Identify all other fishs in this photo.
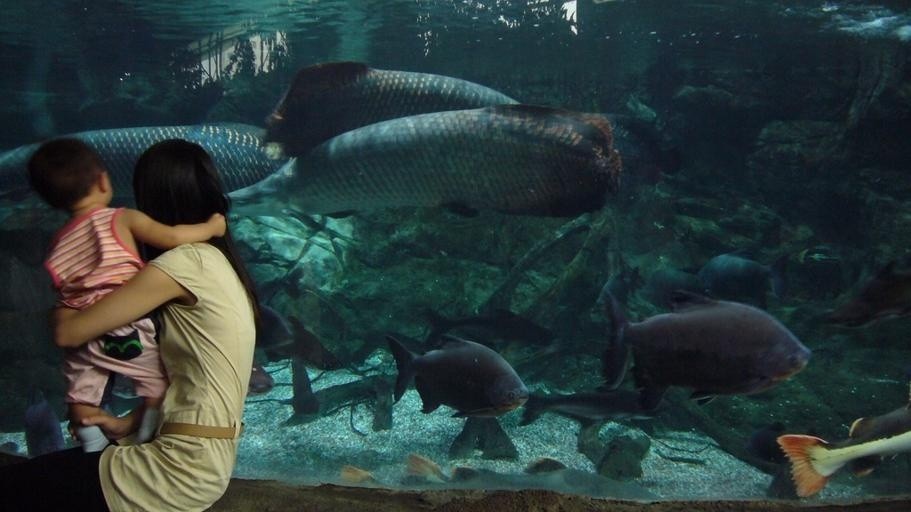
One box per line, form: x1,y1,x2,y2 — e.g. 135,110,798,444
0,60,910,501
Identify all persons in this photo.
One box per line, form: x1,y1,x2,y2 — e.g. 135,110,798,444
27,135,226,453
0,135,260,511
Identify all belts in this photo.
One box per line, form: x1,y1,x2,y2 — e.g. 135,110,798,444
161,422,244,439
